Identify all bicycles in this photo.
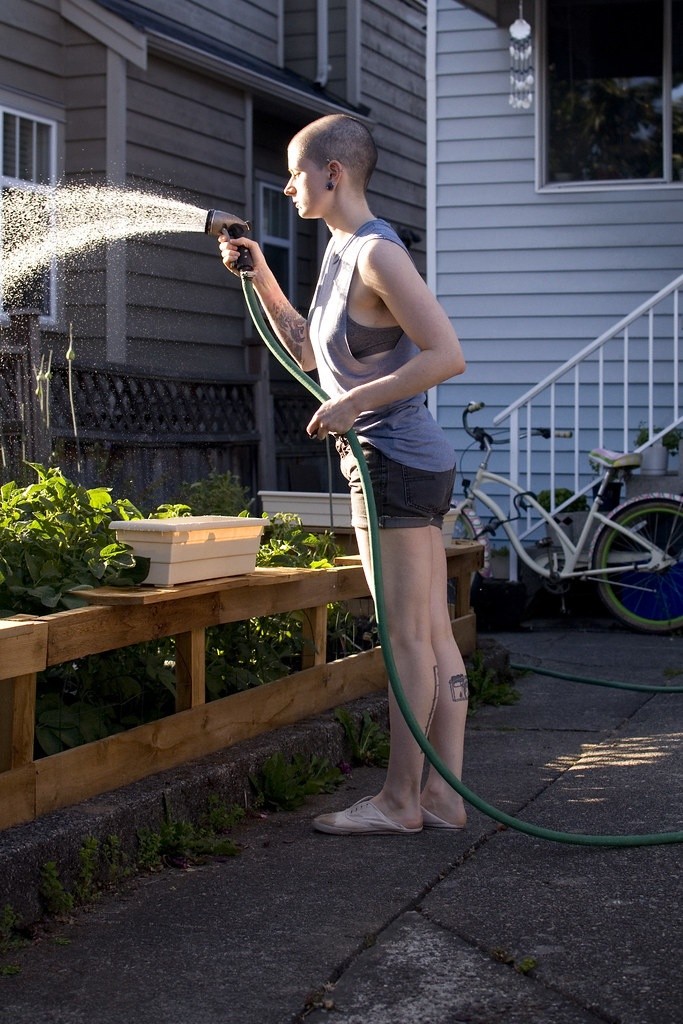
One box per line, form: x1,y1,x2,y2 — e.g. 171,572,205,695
440,402,683,635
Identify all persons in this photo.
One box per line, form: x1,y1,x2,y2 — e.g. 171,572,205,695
218,114,468,835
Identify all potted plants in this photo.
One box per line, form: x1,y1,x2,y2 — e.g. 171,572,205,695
538,488,606,546
634,421,683,476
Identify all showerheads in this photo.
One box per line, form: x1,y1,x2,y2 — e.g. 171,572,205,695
204,208,255,272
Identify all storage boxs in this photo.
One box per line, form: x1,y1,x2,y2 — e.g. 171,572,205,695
257,490,353,527
106,511,271,589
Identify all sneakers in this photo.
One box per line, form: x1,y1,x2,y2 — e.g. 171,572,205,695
312,796,424,834
420,804,467,831
